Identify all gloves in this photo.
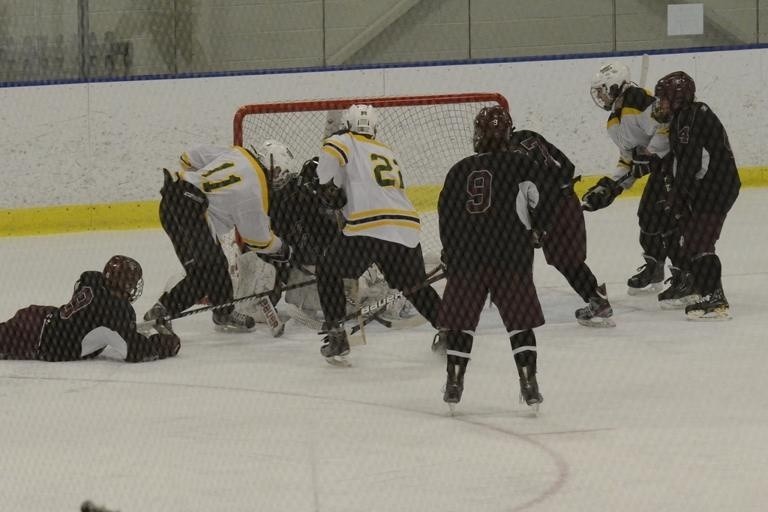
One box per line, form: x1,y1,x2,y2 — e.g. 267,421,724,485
583,176,623,211
630,144,662,178
269,244,294,263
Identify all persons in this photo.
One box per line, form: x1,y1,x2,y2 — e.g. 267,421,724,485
581,59,700,300
510,131,613,321
142,137,295,327
223,157,349,323
1,255,181,363
651,70,741,311
303,103,443,358
438,105,545,405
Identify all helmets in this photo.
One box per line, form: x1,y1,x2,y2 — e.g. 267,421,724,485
105,255,144,304
472,106,513,151
339,103,374,136
651,71,695,123
590,60,630,113
255,141,301,191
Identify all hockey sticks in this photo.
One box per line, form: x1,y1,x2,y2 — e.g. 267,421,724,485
293,273,445,330
293,256,428,329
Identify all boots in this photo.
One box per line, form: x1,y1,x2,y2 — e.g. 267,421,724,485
443,362,465,402
213,307,255,328
518,365,543,405
141,292,182,336
321,320,350,357
576,283,613,319
628,254,729,318
